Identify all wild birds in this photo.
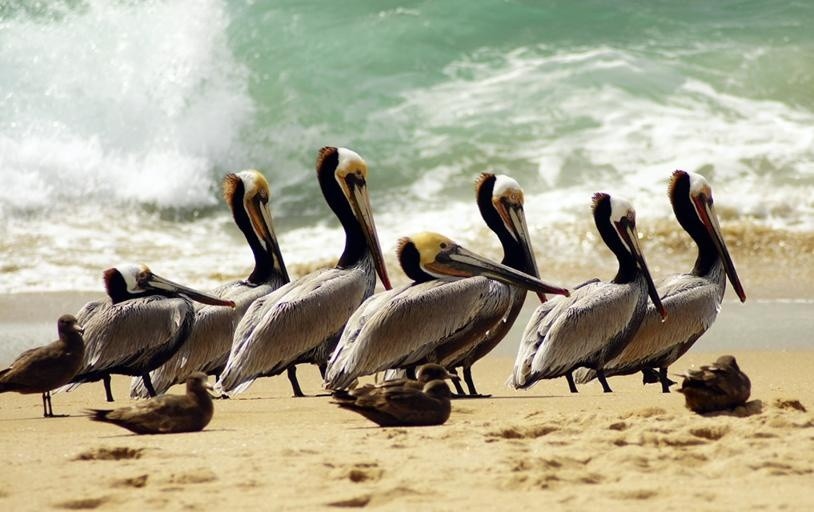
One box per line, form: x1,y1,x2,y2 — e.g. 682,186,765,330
674,351,753,417
323,229,570,392
325,361,462,427
502,190,669,394
0,311,87,421
79,370,215,433
49,260,235,403
571,167,750,392
214,143,395,401
128,167,293,401
417,169,549,401
